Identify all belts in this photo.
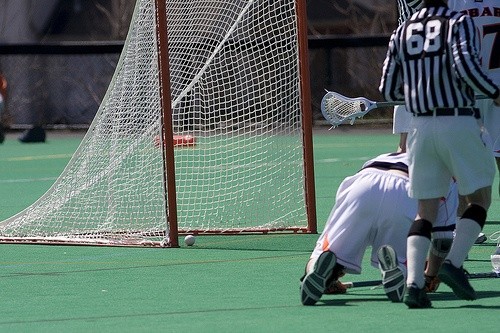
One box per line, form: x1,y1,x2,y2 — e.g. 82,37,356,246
413,107,481,118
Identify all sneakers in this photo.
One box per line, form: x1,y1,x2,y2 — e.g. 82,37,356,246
403,283,433,308
438,259,477,300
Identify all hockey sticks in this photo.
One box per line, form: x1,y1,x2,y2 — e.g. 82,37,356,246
321,88,500,128
338,271,500,289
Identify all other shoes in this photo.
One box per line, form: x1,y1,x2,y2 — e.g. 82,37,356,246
474,233,487,244
376,245,406,304
301,251,337,306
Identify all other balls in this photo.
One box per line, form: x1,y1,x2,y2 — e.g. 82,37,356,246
184,236,195,246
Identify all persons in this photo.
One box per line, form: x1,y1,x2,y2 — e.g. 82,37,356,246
298,0,500,307
